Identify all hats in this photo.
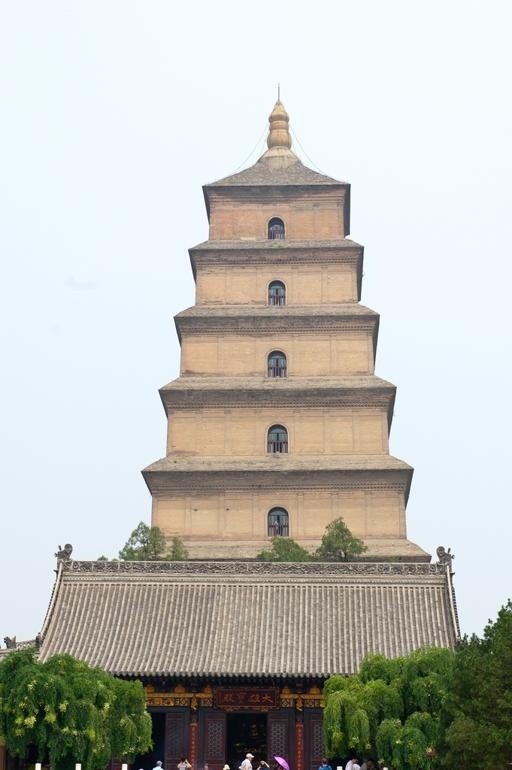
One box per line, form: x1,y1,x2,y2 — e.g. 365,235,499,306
246,753,255,758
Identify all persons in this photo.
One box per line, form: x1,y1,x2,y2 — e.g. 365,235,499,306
152,752,388,770
